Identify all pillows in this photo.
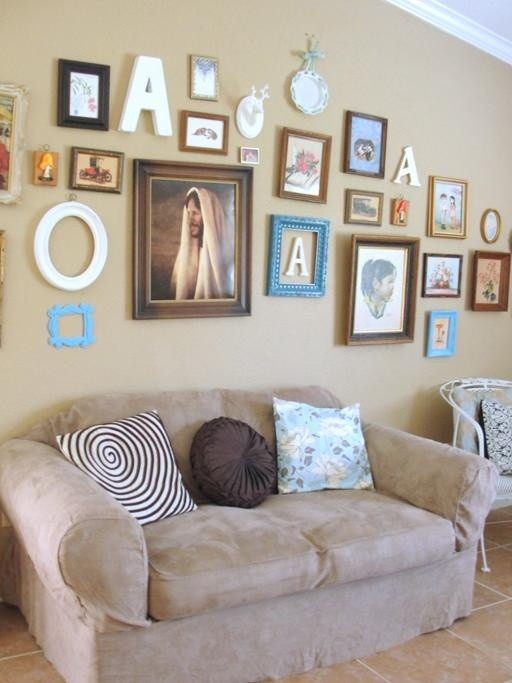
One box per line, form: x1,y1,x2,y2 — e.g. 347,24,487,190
480,399,512,475
189,416,276,508
270,397,375,495
55,409,198,526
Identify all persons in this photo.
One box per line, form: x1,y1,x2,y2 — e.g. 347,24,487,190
361,259,397,320
439,194,471,229
166,185,235,299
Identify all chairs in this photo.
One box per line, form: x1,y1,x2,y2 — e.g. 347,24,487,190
440,378,512,572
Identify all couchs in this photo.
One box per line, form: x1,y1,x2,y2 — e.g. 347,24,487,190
0,384,498,683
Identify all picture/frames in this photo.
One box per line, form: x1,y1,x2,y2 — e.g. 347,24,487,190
187,52,222,104
55,56,112,135
128,156,256,321
425,173,470,242
342,187,385,228
470,248,510,313
237,145,262,165
68,145,126,194
420,251,464,299
423,309,459,357
177,108,232,156
341,108,390,180
343,232,422,348
276,124,334,206
264,212,334,299
0,79,34,204
